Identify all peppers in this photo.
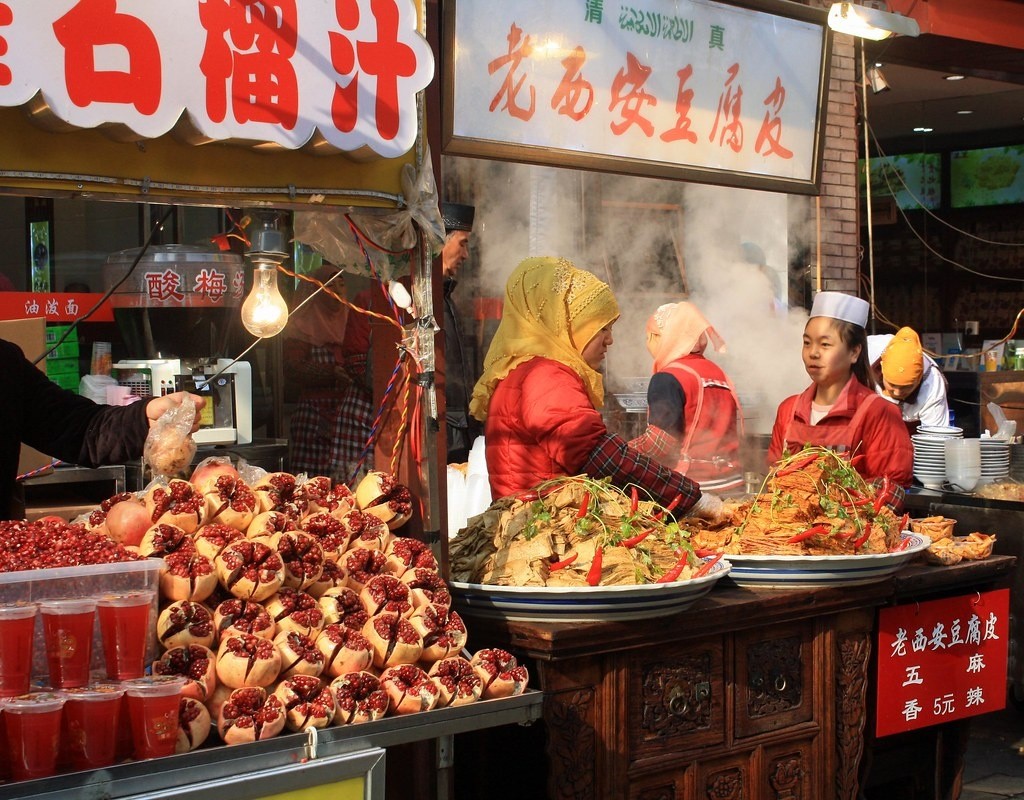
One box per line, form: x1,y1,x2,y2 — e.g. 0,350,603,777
775,452,911,553
513,480,724,582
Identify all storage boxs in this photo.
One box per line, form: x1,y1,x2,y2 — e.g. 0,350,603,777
0,548,165,679
45,326,79,395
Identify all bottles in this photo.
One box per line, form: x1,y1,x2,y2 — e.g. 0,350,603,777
1013,347,1024,372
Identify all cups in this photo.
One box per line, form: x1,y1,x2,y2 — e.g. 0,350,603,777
89,341,113,376
0,590,189,782
106,385,132,406
983,350,997,373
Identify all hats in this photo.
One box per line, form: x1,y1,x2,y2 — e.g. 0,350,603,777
878,326,925,385
807,290,871,330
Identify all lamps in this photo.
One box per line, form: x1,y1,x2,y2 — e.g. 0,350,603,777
827,0,921,42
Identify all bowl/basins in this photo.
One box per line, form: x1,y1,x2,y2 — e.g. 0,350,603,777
910,425,1011,494
907,516,997,567
612,375,651,414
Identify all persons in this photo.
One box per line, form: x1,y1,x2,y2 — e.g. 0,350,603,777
865,326,949,436
765,291,914,516
626,300,746,501
282,263,374,492
440,202,484,465
469,256,740,525
0,337,207,521
0,273,129,377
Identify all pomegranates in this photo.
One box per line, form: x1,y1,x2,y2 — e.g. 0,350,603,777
0,427,528,754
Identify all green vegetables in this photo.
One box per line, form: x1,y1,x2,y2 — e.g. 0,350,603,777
522,473,695,583
734,440,891,550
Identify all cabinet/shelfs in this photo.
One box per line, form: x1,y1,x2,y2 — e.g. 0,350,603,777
940,368,1024,437
460,556,1018,800
855,132,1024,336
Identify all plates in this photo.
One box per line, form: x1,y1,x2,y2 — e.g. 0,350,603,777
449,555,733,623
714,529,933,590
